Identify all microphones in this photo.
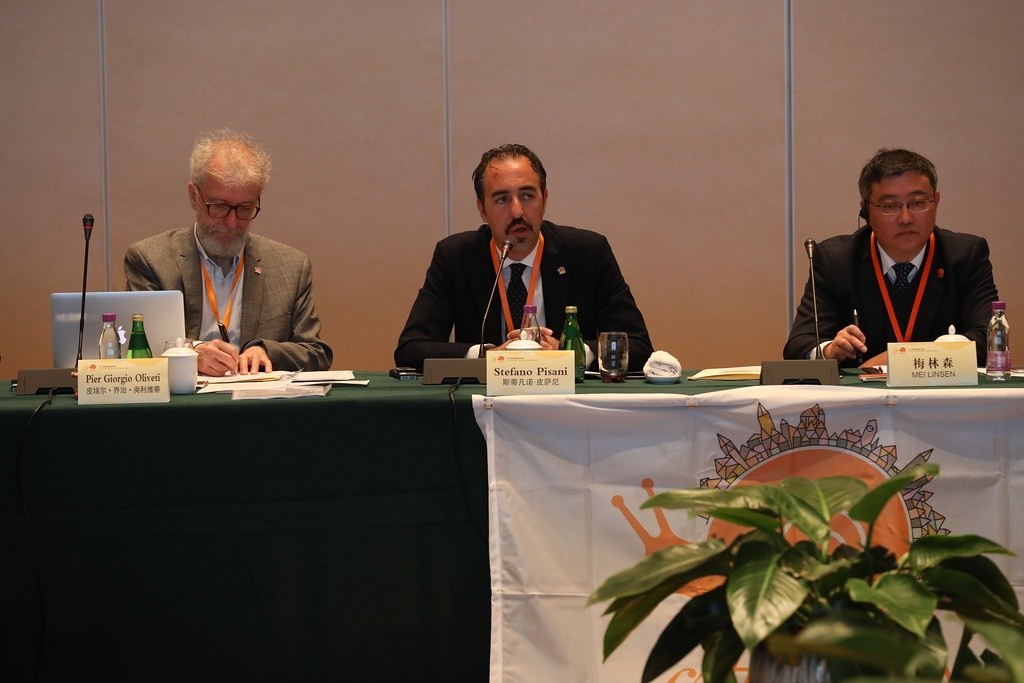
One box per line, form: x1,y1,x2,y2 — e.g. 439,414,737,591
478,239,513,358
805,238,824,360
76,213,94,369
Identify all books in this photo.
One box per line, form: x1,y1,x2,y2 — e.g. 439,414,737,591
859,365,887,382
196,370,371,400
687,366,761,380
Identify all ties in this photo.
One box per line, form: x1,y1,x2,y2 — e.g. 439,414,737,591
505,261,528,341
891,261,915,295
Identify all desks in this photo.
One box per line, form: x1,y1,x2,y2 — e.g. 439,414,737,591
1,370,1024,683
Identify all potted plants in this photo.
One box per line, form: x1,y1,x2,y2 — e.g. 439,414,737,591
580,463,1024,683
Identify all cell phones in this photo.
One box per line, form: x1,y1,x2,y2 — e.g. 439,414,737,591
390,369,419,380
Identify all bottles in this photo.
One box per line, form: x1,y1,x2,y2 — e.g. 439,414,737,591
518,305,541,345
985,301,1012,380
557,305,586,384
125,313,152,358
98,313,121,359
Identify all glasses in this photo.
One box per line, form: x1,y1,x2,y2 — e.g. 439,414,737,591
867,195,935,215
194,183,261,220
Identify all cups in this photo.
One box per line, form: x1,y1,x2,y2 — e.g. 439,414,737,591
933,325,971,343
505,330,542,351
161,338,199,394
597,331,629,383
164,337,193,351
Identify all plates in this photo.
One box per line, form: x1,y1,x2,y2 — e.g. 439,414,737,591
646,375,681,384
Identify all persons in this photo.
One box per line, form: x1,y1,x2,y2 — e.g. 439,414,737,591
393,145,654,376
782,149,999,368
123,127,333,377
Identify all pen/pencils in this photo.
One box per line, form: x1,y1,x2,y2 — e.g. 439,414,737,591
218,323,237,374
853,309,863,363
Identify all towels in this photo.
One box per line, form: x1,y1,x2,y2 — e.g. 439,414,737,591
642,349,682,377
